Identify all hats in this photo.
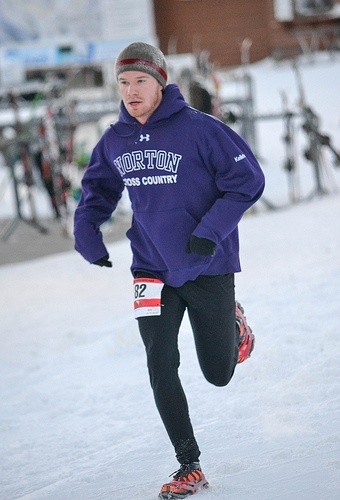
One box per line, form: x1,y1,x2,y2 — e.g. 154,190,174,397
115,42,168,89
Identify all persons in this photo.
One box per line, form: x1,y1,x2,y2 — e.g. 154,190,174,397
72,43,265,500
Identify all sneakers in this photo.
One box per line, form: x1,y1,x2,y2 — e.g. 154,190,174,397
235,300,254,363
159,462,208,499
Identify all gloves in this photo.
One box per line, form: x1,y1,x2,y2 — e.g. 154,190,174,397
94,255,112,268
185,236,216,256
